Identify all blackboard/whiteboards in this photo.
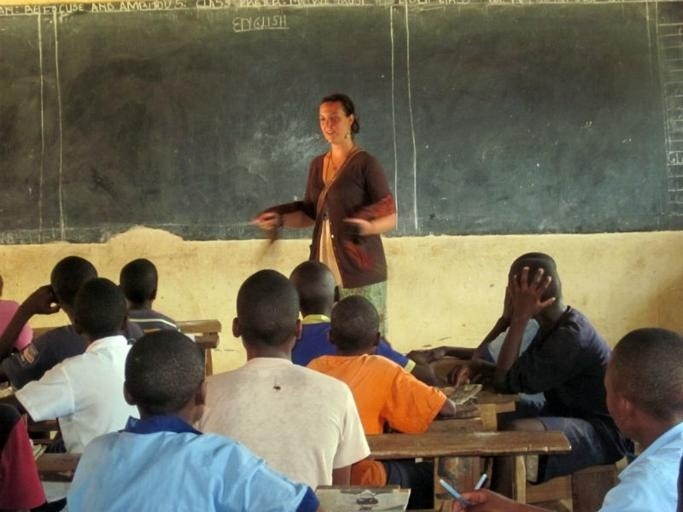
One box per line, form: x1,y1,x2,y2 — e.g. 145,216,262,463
0,0,681,245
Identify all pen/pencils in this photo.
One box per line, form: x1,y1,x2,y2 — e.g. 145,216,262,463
434,476,461,498
473,471,491,491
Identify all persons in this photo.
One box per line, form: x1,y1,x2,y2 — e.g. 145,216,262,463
60,328,325,512
189,268,372,490
1,255,147,391
11,277,141,454
438,327,683,512
449,252,632,485
426,250,559,367
252,93,398,339
303,295,457,509
120,257,183,344
1,276,34,351
0,402,52,511
289,259,440,387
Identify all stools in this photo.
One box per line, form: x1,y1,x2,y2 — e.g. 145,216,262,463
574,455,628,497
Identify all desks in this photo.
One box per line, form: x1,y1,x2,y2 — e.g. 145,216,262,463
30,327,59,350
364,433,572,504
0,382,63,446
436,385,513,433
35,453,82,482
310,485,412,512
175,320,222,380
